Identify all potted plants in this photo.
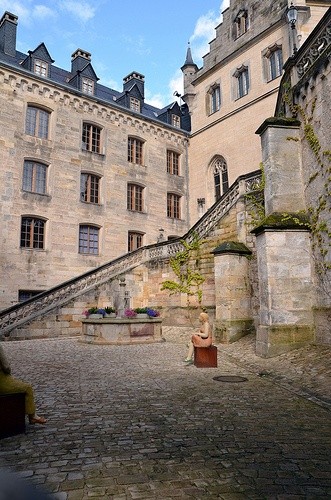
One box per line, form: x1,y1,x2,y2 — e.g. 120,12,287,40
104,306,116,319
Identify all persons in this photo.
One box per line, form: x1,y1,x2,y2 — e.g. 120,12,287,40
0,342,47,425
183,313,212,362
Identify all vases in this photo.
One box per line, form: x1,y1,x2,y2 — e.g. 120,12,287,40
136,313,149,319
89,314,103,319
150,315,159,319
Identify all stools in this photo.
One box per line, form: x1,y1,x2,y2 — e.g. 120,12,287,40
0,390,28,437
194,344,217,368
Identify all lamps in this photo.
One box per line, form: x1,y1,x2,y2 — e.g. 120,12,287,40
286,1,298,30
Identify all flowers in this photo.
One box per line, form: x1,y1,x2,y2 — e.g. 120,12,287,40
83,307,105,316
117,307,160,317
